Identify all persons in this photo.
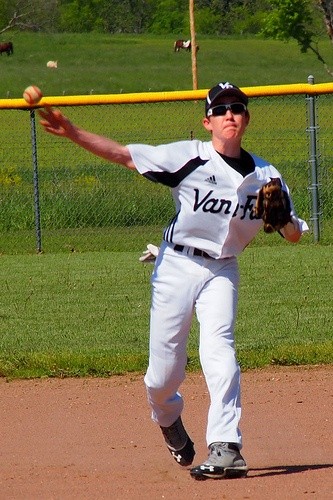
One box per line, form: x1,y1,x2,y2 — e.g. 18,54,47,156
38,81,310,482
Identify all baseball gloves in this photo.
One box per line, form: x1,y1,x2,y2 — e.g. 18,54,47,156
253,178,291,233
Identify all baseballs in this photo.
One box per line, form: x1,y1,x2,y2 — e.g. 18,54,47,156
23,86,42,104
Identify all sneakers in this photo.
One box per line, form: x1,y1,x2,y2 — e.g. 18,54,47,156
190,442,248,478
158,416,194,467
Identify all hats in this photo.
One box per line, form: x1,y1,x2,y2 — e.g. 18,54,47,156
205,81,248,115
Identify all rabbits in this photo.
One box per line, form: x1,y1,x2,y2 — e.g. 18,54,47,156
46,55,59,69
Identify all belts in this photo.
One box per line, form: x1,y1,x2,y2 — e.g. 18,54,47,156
174,244,209,258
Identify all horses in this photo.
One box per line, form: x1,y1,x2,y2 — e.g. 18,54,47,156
0,41,14,56
174,39,191,53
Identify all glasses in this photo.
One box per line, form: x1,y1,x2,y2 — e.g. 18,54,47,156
207,103,247,117
